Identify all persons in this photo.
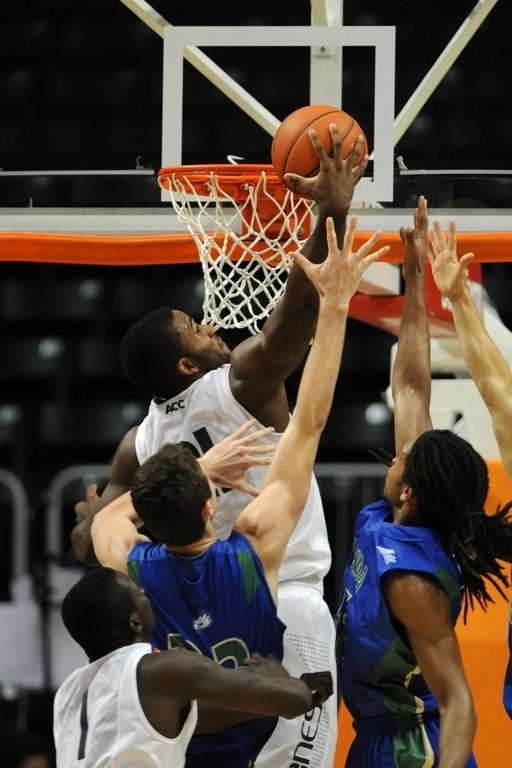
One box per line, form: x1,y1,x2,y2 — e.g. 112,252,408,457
423,214,511,717
70,123,371,767
90,214,390,768
52,566,313,768
334,192,511,768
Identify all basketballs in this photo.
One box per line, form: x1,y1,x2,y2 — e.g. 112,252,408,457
271,105,368,199
